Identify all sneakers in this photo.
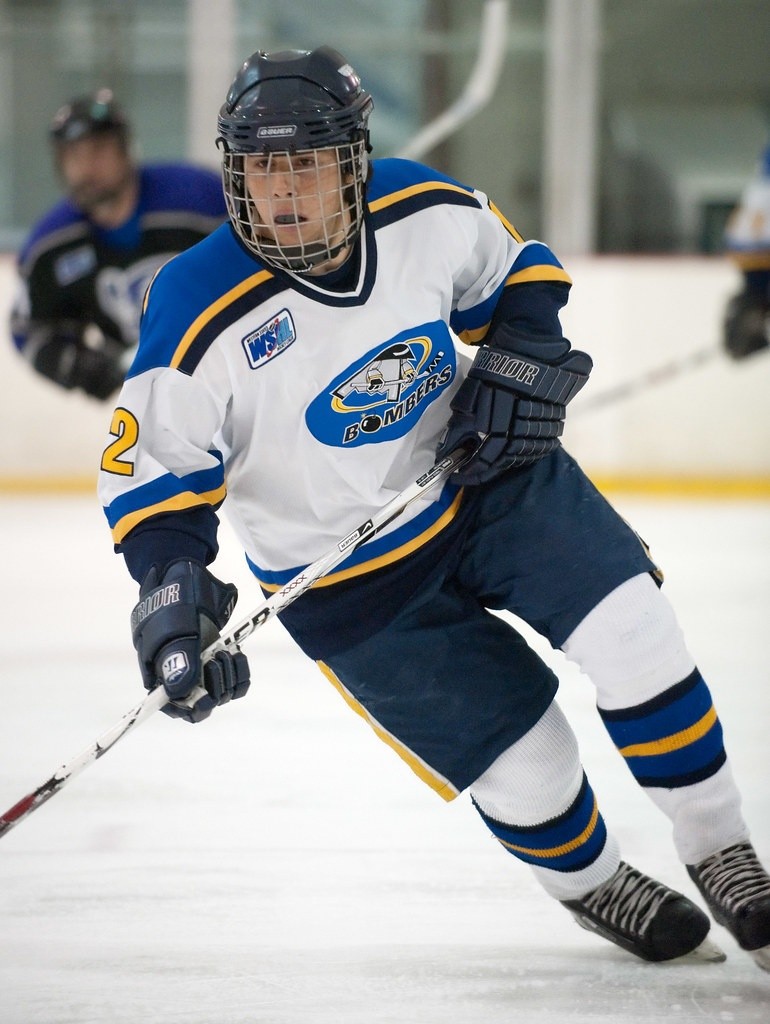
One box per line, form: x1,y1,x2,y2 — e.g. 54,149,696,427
558,860,729,964
685,839,769,974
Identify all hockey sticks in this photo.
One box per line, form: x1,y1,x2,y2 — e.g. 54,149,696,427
567,337,727,421
0,431,488,839
118,0,507,371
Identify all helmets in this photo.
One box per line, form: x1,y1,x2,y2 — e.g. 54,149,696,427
214,43,375,275
47,89,135,161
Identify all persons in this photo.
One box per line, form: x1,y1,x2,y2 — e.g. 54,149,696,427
10,93,230,401
98,45,770,969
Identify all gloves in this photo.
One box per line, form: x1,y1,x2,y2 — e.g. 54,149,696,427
431,327,593,487
130,559,252,725
